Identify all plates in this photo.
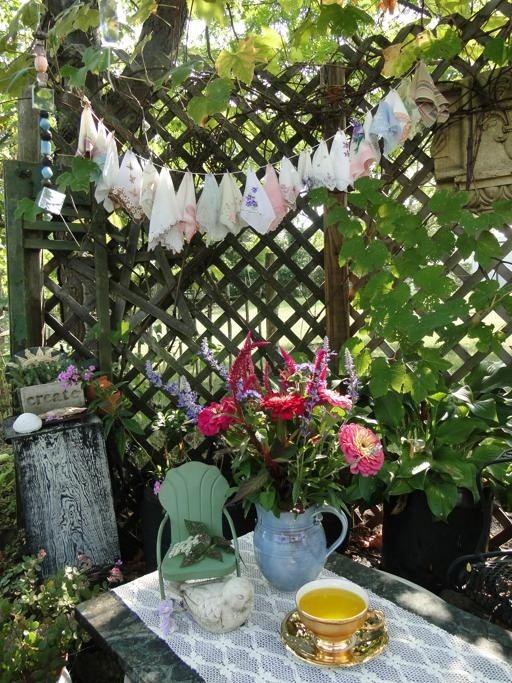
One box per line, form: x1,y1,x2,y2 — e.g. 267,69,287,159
281,608,389,668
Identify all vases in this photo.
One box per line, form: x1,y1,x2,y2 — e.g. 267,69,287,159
13,379,84,415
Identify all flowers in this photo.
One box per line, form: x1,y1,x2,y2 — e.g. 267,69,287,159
139,336,386,507
6,346,67,388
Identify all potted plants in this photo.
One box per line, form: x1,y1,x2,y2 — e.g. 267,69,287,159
303,168,512,591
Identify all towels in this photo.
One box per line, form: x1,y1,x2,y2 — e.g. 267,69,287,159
73,60,457,252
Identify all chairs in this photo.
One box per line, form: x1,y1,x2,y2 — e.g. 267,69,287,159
443,549,511,632
148,459,247,604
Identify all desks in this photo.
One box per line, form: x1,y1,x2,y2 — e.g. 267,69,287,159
71,528,512,683
2,410,123,583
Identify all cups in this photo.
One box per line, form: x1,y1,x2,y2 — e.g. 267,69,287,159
295,577,386,655
